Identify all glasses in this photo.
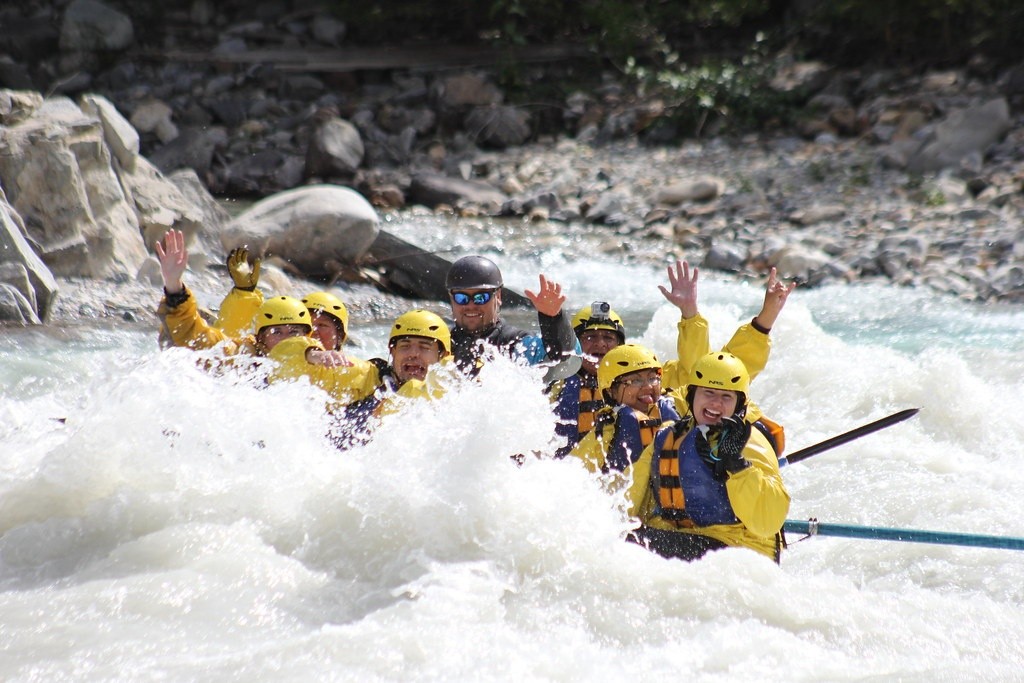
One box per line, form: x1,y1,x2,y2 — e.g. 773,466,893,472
450,289,498,305
614,376,662,387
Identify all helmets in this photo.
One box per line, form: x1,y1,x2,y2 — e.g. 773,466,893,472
685,352,749,416
388,311,452,368
597,345,663,405
446,256,503,289
255,296,313,337
301,292,347,346
571,306,625,345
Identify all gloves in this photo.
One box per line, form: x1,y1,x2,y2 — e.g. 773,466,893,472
716,413,752,474
227,245,260,291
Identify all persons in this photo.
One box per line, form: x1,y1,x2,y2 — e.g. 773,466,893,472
211,245,349,351
609,350,791,564
155,228,313,384
445,255,584,383
545,260,710,475
264,308,470,452
562,266,796,527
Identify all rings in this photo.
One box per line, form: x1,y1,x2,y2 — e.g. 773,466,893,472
553,290,561,296
